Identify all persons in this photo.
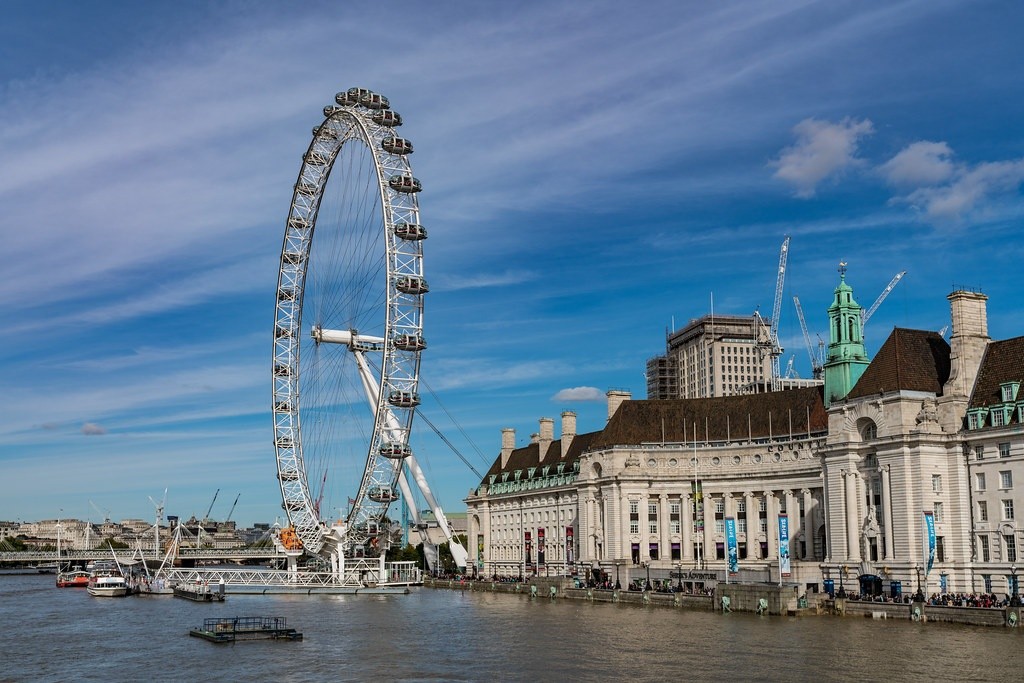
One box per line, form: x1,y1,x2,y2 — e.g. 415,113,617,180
825,591,1024,611
205,579,209,593
436,573,529,582
574,580,715,595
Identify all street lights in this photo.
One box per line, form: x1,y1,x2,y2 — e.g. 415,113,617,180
836,562,847,599
1010,563,1022,607
674,563,683,592
913,563,924,602
645,563,652,591
615,561,622,589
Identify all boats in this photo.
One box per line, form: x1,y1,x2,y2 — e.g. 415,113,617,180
56,565,90,589
88,561,127,598
